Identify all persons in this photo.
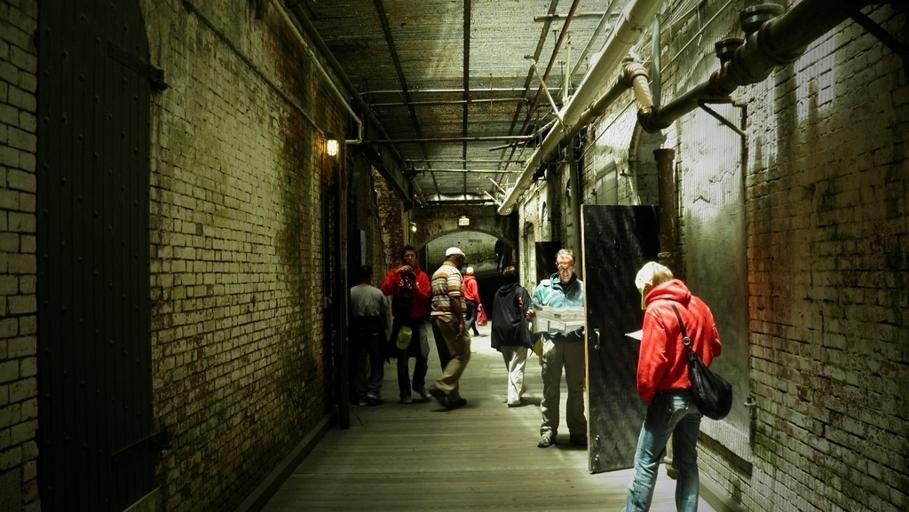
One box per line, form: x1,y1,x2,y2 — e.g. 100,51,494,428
381,246,432,404
527,247,587,447
463,267,481,337
623,261,723,511
350,263,390,406
492,263,542,408
431,247,474,408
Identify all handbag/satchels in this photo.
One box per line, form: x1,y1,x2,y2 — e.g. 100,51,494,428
477,306,487,325
684,335,732,420
515,319,534,347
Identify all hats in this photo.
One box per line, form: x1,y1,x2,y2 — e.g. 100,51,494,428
635,261,662,310
445,247,465,256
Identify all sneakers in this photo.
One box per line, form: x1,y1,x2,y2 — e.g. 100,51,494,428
402,396,412,403
537,430,556,447
359,389,377,405
430,387,451,407
412,385,432,399
450,397,467,409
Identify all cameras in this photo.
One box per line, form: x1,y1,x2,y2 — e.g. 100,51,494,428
401,270,416,286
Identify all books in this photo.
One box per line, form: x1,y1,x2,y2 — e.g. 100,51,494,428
623,329,643,342
